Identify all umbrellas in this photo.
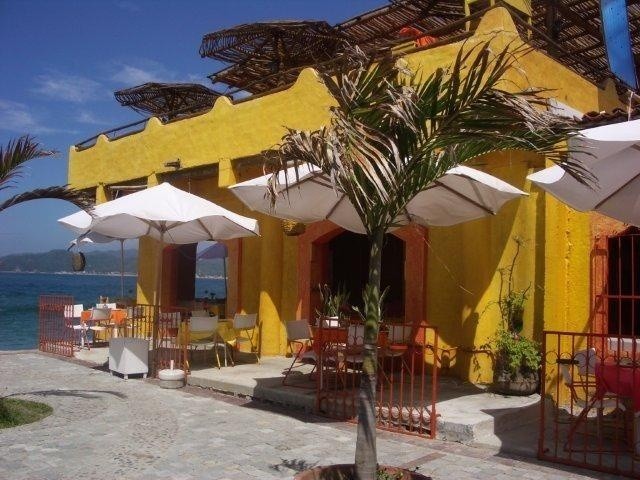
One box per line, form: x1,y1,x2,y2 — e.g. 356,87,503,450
65,230,129,304
57,182,260,378
196,241,229,297
525,118,640,228
227,150,529,235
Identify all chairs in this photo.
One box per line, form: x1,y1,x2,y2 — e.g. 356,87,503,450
156,310,260,370
63,298,144,351
557,346,627,453
281,314,416,394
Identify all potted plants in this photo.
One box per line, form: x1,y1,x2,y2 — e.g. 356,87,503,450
467,232,544,396
